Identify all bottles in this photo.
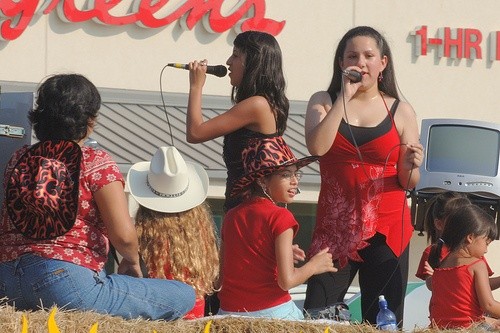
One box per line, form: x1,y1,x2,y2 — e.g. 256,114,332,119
376,296,396,333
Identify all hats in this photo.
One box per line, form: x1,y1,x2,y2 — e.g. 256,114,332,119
7,140,82,239
127,146,209,213
227,136,322,197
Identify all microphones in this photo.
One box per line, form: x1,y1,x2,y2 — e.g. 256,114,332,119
168,63,227,77
342,69,361,82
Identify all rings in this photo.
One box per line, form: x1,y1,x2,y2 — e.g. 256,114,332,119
199,62,208,66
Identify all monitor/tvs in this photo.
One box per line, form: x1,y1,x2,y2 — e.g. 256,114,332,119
415,118,500,200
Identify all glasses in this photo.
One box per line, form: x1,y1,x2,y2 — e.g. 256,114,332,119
270,169,303,179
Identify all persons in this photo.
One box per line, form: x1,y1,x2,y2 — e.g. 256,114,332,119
0,73,196,321
186,31,289,214
117,144,224,320
428,203,500,331
303,25,424,333
216,135,338,321
415,190,500,292
